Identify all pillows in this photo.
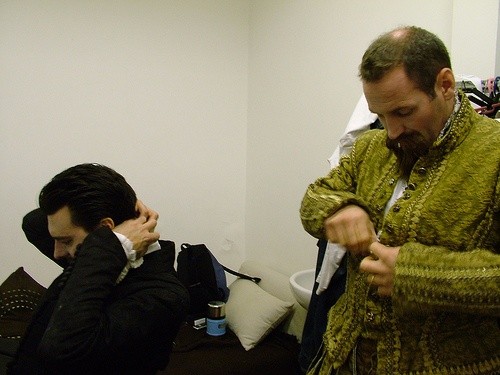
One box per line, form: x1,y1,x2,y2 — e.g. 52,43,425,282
222,270,295,351
0,267,48,358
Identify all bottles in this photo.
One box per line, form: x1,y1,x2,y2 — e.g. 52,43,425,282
206,301,227,336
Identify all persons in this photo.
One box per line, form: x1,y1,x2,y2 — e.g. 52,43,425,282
298,26,500,375
3,162,188,375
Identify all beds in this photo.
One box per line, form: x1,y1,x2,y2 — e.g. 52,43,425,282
0,307,302,375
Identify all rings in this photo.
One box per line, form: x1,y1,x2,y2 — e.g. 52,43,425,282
371,273,375,284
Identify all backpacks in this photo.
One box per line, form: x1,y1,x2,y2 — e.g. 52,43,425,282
177,243,262,314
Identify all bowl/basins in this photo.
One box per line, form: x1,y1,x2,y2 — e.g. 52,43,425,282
290,270,315,308
